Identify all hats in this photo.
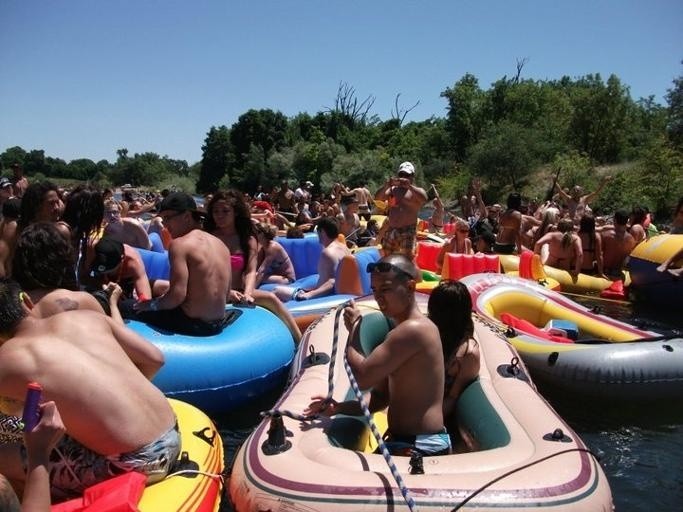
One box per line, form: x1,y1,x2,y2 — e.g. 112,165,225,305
90,239,125,272
159,192,208,218
121,184,132,188
397,161,415,177
0,177,13,189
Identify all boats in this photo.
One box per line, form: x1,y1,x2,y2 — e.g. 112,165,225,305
459,271,683,403
231,290,614,511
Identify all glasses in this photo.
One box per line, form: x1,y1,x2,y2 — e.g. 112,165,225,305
367,262,414,279
399,172,411,177
107,210,120,215
161,210,185,225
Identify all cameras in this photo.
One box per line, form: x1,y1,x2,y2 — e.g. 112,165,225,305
391,177,400,186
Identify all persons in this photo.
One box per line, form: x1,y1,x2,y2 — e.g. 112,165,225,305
1,160,682,512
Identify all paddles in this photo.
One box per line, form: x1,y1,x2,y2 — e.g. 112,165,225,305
373,200,387,210
545,166,561,202
